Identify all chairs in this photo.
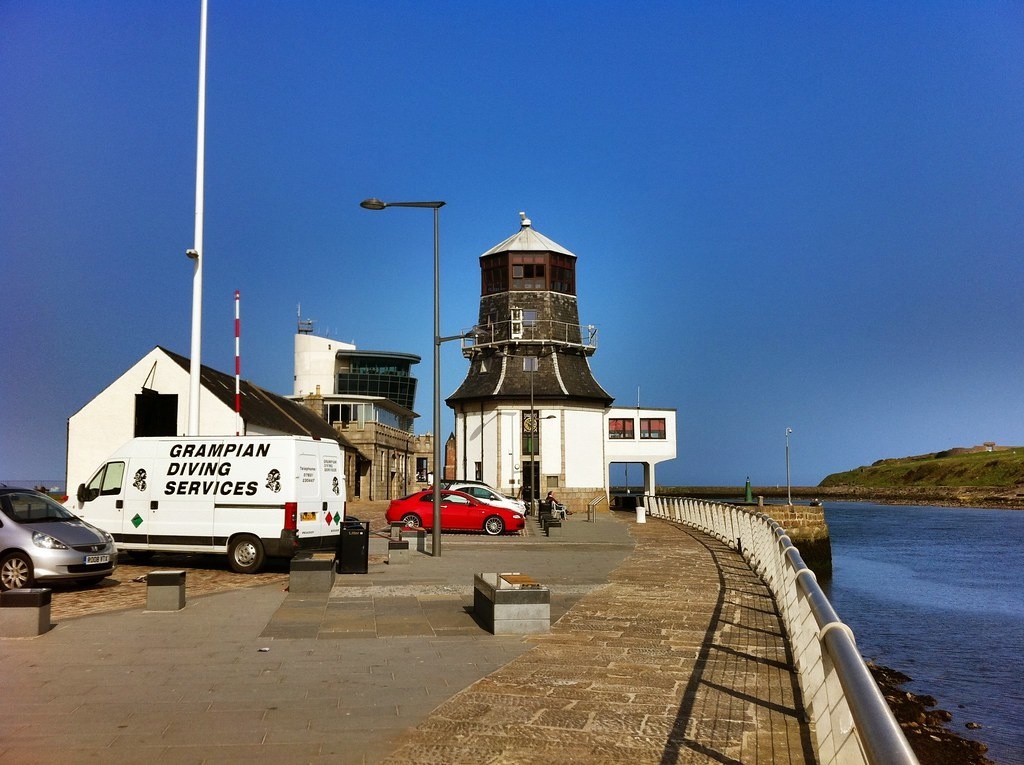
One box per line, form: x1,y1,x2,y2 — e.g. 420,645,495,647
551,501,567,521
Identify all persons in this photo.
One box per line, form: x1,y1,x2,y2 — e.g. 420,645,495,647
545,491,573,520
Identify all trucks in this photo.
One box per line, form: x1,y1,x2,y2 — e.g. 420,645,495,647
59,437,346,573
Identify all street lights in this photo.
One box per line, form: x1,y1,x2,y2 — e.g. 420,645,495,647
528,415,556,516
179,243,205,437
361,195,491,557
786,426,794,506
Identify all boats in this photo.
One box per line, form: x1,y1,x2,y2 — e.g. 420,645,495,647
810,498,822,506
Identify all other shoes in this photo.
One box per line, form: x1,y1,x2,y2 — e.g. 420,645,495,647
567,511,573,515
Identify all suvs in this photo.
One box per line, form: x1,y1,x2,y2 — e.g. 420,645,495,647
423,478,495,491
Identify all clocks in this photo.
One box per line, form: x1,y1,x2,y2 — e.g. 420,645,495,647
524,418,537,430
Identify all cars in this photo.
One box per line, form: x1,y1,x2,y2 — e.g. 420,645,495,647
0,478,120,594
445,484,527,517
385,490,526,535
